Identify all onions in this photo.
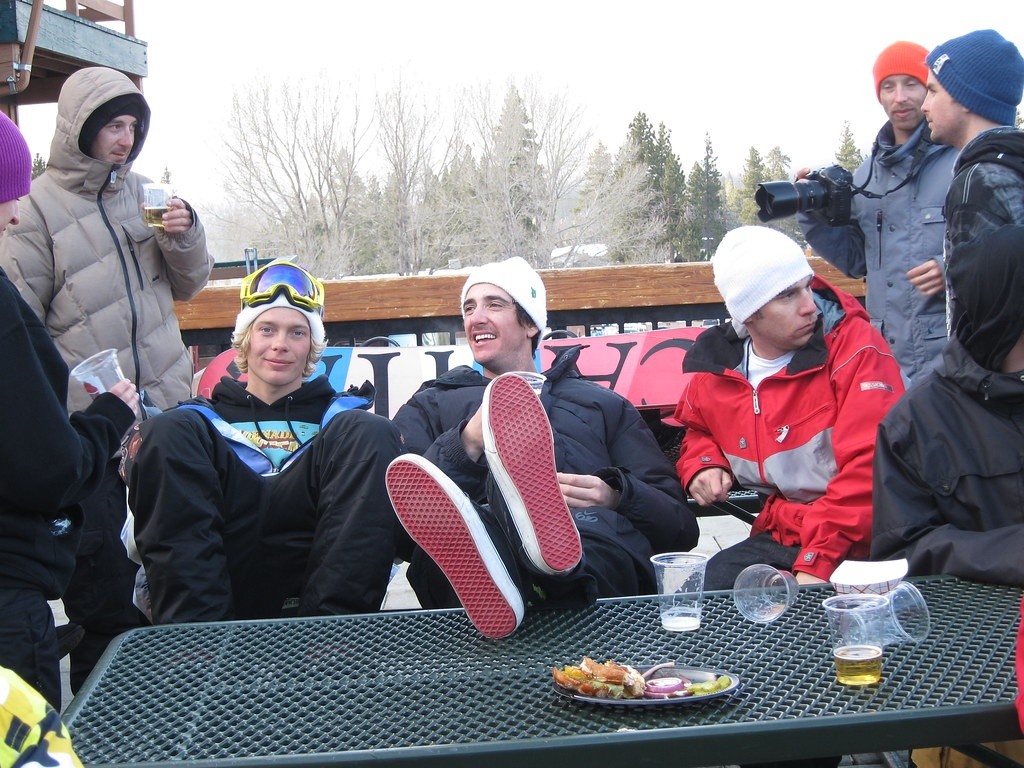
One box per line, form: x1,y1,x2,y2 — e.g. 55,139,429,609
644,677,694,697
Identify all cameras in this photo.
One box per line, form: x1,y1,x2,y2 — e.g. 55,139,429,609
754,164,853,226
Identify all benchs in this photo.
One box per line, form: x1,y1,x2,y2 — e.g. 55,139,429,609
198,331,764,524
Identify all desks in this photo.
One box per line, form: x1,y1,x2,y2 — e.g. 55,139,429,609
60,577,1024,768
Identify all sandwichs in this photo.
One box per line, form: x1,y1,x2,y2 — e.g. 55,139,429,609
553,657,646,700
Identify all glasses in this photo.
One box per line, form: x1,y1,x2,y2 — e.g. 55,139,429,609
240,255,326,312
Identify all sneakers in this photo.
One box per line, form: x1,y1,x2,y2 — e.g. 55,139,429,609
482,373,583,575
386,453,524,640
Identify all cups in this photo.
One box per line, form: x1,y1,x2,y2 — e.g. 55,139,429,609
650,552,709,631
143,182,174,227
733,563,800,623
506,370,547,401
69,348,125,400
839,580,930,647
822,594,890,685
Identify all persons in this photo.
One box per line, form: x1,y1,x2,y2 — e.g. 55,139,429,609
384,256,701,640
118,256,407,627
796,27,1024,739
0,65,215,698
675,225,907,768
0,109,139,712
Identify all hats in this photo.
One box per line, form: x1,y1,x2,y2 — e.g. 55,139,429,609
238,297,327,345
928,29,1023,125
875,41,929,102
461,256,547,332
78,94,143,155
0,113,31,204
712,227,814,322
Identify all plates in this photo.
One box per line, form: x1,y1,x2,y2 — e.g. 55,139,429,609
550,664,739,704
